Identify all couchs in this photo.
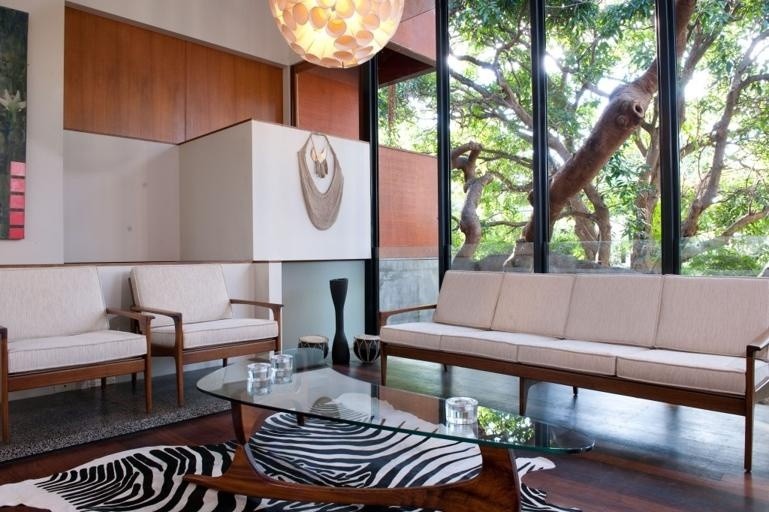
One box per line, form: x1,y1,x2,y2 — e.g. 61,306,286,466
370,264,769,473
121,261,283,406
0,264,155,439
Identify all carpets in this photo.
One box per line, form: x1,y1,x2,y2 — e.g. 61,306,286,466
0,408,585,511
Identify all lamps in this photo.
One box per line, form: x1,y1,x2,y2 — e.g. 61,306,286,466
269,0,408,68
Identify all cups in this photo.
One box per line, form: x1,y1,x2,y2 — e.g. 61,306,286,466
247,362,275,396
268,354,295,384
446,423,480,439
444,396,478,423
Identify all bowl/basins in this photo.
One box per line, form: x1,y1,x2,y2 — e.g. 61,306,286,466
283,348,324,369
